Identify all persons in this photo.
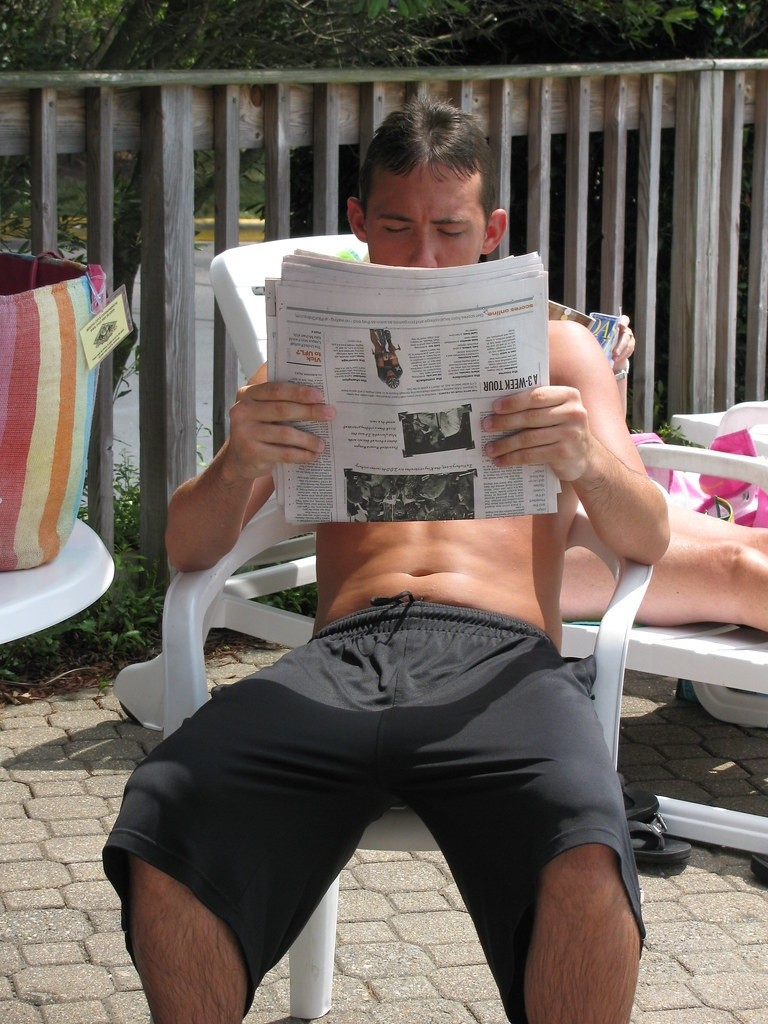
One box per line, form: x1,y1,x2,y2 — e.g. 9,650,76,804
102,101,768,1024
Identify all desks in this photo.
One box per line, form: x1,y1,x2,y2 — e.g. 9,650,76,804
0,516,115,643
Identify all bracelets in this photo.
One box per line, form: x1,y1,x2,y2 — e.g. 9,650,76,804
615,358,630,381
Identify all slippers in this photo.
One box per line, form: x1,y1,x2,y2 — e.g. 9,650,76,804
623,814,691,865
618,772,660,821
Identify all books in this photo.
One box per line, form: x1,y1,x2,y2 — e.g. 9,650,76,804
547,298,622,358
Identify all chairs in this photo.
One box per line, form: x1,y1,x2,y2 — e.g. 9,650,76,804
161,483,652,1017
113,233,768,854
